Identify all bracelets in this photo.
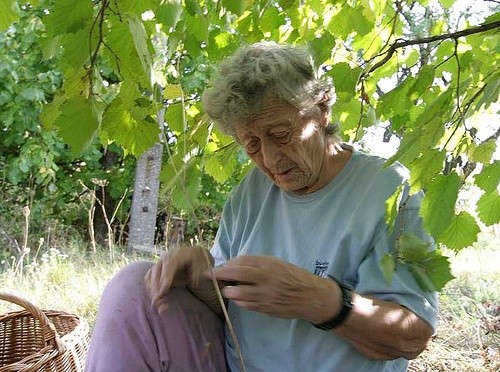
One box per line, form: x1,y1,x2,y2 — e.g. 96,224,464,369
311,273,354,331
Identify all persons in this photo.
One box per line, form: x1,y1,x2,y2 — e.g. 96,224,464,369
82,40,441,372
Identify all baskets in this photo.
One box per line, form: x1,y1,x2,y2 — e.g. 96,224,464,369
0,293,89,371
